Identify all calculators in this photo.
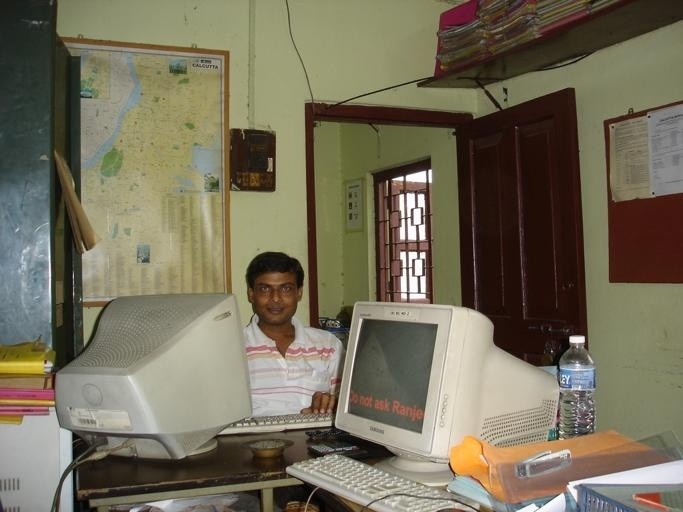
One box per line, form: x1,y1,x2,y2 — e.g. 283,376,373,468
308,439,369,459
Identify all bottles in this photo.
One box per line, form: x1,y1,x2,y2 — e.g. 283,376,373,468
559,335,597,441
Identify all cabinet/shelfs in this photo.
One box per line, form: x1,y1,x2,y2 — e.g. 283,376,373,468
0,0,84,387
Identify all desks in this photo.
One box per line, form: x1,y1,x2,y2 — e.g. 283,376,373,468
77,428,360,512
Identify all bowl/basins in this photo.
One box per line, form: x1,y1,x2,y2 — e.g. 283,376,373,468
239,438,294,458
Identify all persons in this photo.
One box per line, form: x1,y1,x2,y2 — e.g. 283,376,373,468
243,252,345,414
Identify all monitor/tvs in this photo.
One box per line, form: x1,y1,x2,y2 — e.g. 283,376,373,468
54,293,253,461
335,300,561,486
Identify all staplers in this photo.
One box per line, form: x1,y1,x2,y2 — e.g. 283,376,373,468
513,449,572,480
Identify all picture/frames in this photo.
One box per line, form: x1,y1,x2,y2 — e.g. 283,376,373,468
57,35,231,308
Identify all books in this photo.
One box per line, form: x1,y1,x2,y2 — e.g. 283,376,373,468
0,342,56,425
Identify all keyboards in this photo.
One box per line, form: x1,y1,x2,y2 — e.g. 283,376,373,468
217,412,335,435
284,453,481,512
